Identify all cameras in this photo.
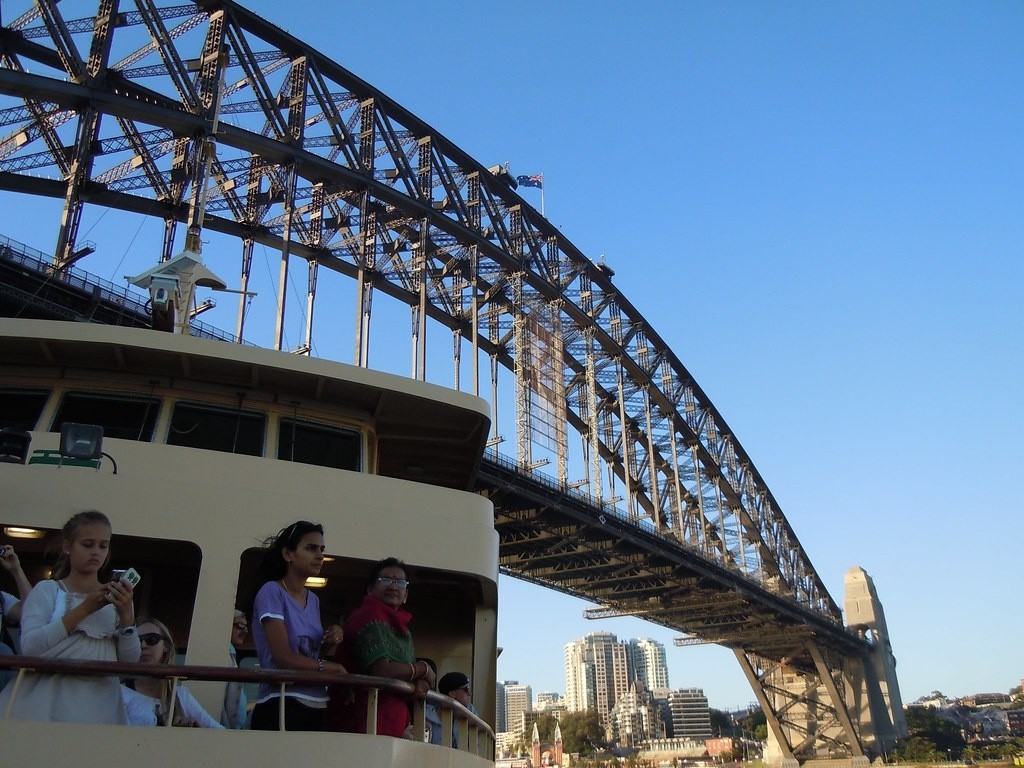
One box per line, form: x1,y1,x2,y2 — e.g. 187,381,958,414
0,547,5,559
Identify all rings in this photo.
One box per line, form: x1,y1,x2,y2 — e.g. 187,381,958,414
335,637,339,640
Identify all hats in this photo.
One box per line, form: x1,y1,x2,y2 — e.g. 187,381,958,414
438,672,469,693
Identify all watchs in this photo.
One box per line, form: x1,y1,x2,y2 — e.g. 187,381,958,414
119,623,137,637
317,655,324,673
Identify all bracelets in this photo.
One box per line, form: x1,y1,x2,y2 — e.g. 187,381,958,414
414,663,419,679
410,663,415,679
419,660,429,678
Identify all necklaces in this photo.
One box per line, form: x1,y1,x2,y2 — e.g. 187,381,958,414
280,579,308,609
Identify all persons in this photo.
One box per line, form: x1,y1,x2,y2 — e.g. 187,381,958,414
0,510,479,748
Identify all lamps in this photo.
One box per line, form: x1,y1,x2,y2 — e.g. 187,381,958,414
59,422,103,471
492,163,518,191
597,263,615,276
0,427,32,465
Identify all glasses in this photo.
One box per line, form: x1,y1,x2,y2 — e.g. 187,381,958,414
233,622,250,631
378,577,410,588
459,684,471,693
138,632,167,646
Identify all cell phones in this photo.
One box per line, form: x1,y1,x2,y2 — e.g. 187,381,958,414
111,570,127,584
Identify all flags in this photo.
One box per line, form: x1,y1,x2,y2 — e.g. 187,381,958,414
517,175,542,189
